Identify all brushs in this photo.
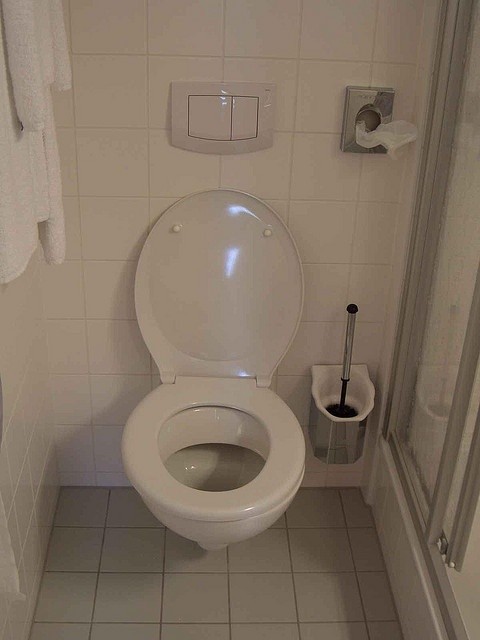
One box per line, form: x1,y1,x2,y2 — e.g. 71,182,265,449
336,303,360,413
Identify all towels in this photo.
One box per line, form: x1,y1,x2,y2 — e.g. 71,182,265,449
1,2,74,285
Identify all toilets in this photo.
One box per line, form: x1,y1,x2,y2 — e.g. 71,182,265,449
120,187,306,544
